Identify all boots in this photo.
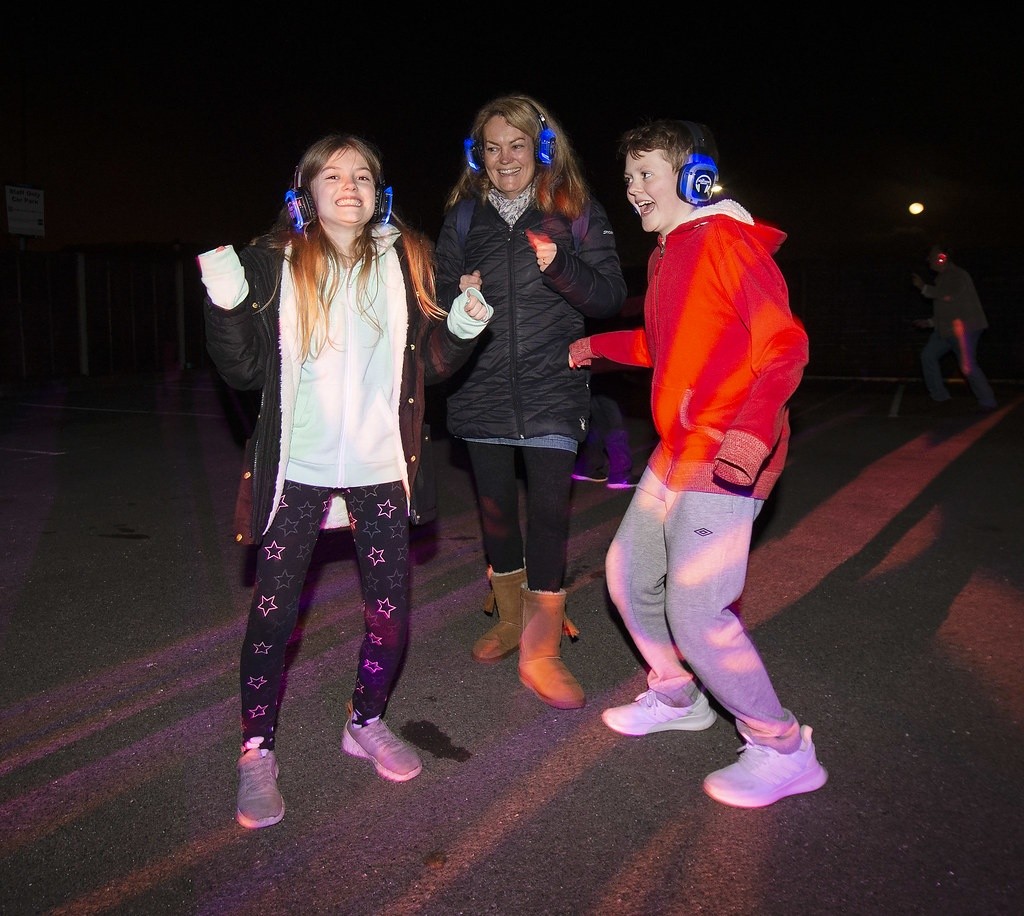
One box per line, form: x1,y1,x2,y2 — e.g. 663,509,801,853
472,565,529,663
519,583,586,710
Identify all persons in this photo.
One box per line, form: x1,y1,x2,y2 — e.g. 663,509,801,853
912,243,999,413
195,135,496,831
434,95,629,709
572,395,640,488
568,120,829,811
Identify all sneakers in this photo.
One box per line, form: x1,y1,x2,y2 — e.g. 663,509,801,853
704,724,828,808
236,747,284,829
601,690,718,737
341,714,423,782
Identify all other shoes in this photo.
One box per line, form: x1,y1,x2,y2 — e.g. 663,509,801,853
968,400,1000,413
918,397,953,409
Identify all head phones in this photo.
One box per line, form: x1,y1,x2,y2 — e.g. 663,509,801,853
463,97,555,178
285,153,393,234
934,243,949,266
676,120,719,207
